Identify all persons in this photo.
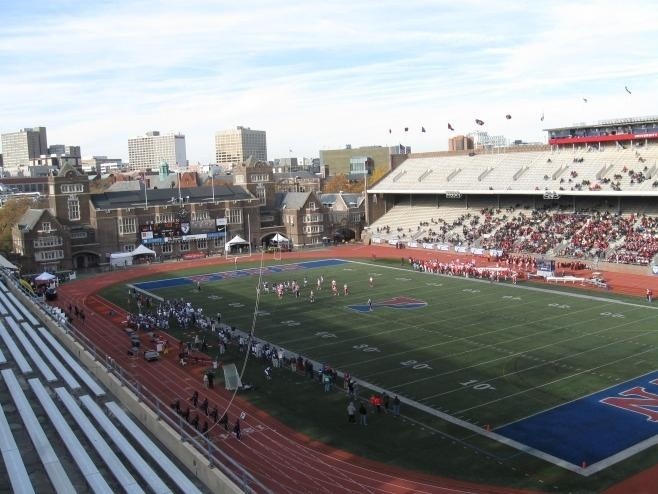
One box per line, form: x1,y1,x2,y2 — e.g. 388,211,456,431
535,157,657,191
264,274,375,311
135,253,161,265
173,398,209,438
127,286,248,386
415,202,657,266
68,304,85,322
58,272,67,284
193,391,242,438
319,363,338,393
250,340,314,381
400,257,413,266
343,369,400,427
411,251,583,285
324,234,345,245
647,288,653,302
27,278,49,297
377,224,412,249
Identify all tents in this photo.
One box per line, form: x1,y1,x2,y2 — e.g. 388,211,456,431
228,235,251,256
272,232,290,250
35,272,57,288
133,244,156,260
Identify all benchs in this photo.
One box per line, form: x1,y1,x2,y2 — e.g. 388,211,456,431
1,281,206,493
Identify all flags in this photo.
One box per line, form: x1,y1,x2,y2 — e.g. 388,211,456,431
388,114,512,134
